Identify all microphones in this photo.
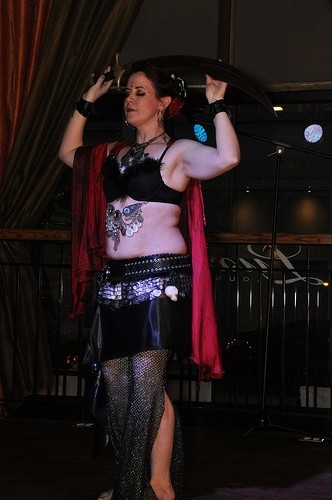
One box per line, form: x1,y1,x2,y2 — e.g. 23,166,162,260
88,53,152,91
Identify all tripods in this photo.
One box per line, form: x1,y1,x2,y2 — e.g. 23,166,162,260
216,145,332,453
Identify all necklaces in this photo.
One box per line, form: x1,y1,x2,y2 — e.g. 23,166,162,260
121,130,167,163
76,97,94,119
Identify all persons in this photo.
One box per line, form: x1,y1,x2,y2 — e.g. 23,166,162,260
58,58,241,500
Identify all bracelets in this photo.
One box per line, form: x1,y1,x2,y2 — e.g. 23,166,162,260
208,99,228,120
